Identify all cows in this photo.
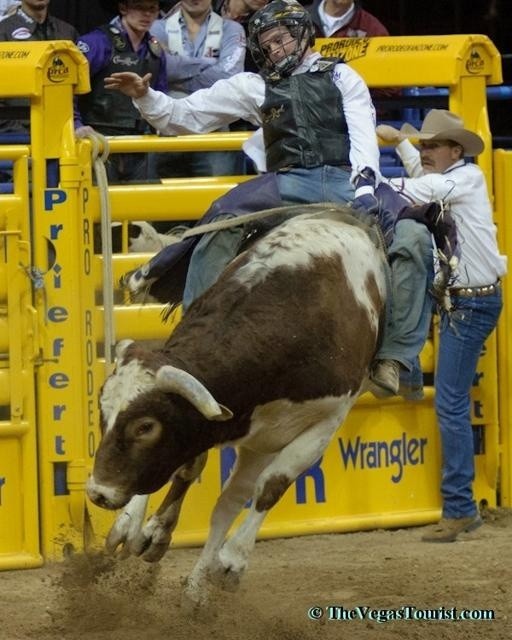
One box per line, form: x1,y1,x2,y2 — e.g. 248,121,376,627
82,206,395,619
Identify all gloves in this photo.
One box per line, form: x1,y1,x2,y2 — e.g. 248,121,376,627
353,180,379,219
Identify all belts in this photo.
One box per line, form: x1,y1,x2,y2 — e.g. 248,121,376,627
449,278,504,299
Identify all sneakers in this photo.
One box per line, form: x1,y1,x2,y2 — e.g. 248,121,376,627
370,350,402,397
421,508,483,543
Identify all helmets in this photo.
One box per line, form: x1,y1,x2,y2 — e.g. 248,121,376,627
399,104,484,158
244,0,318,86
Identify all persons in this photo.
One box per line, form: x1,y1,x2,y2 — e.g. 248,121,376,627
104,0,434,396
375,109,507,544
